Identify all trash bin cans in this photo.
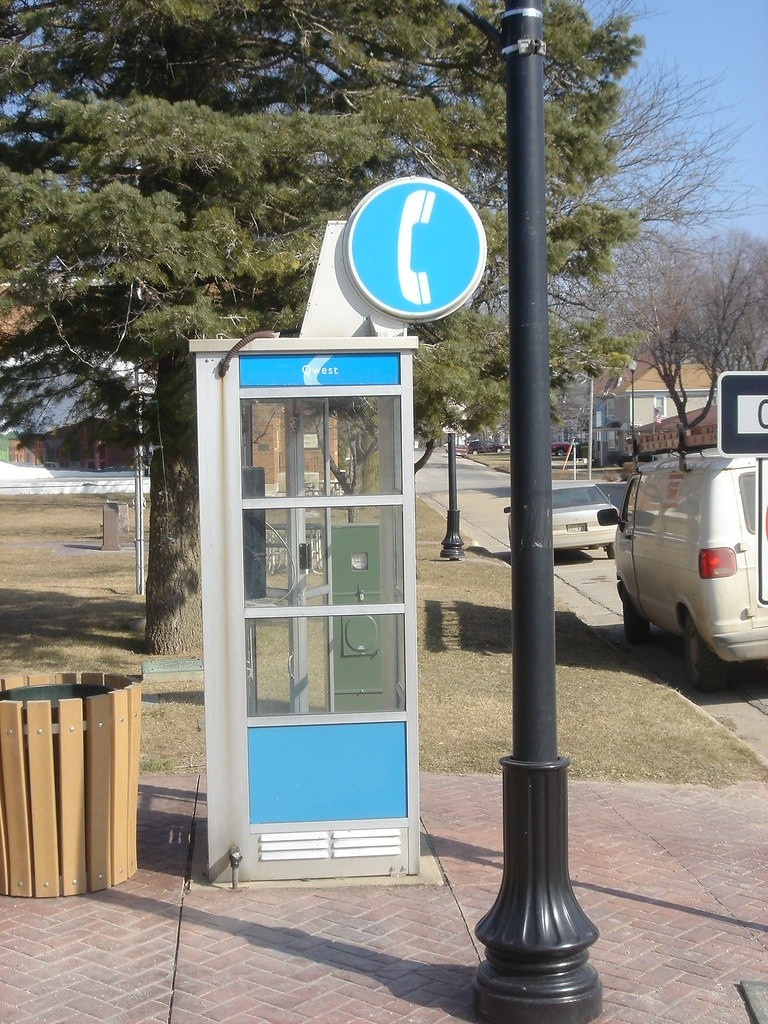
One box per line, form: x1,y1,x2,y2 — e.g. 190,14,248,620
0,668,146,907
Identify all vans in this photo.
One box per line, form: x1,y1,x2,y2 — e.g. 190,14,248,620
595,456,768,695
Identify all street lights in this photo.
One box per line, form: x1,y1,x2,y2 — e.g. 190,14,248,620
629,359,637,456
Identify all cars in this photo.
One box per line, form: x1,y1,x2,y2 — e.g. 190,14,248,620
444,437,467,451
468,440,505,455
503,480,621,559
551,436,571,457
455,444,467,458
